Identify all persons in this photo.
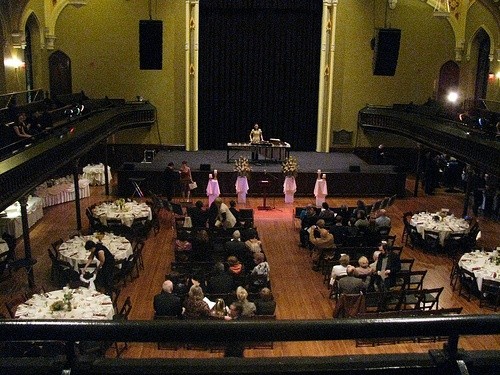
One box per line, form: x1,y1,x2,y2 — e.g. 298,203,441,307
330,241,405,302
309,225,337,272
249,123,264,162
153,228,276,321
13,106,53,141
180,161,193,202
79,270,97,291
418,145,463,195
161,162,181,201
176,197,239,260
298,200,391,249
81,240,121,297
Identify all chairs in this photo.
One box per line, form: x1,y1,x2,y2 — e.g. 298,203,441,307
293,194,500,347
0,191,276,358
0,91,113,162
392,100,500,142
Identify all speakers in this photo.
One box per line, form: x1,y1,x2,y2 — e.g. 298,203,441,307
200,164,211,171
138,20,162,70
373,29,401,76
349,166,360,173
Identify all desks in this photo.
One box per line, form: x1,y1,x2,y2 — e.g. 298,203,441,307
57,234,133,274
0,238,9,268
14,289,115,344
227,142,290,164
66,165,112,186
410,212,469,246
0,197,43,239
128,177,145,200
34,178,90,207
458,252,500,291
91,202,152,227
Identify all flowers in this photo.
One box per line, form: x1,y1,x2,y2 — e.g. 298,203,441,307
281,156,299,176
234,155,250,177
